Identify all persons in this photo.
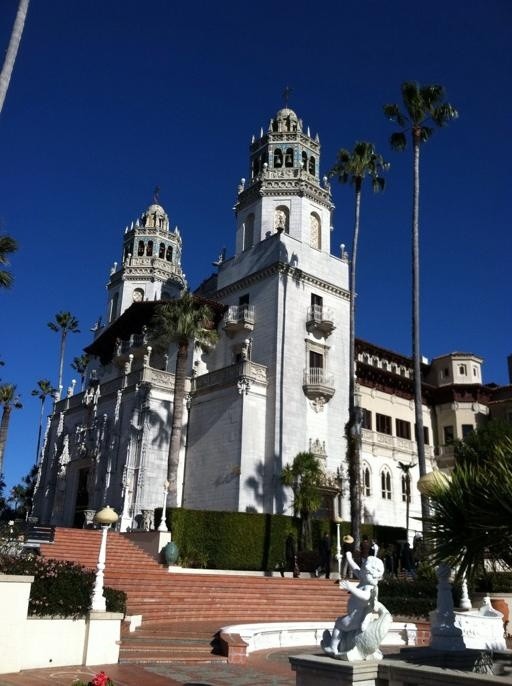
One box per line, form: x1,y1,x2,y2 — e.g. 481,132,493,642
385,552,399,579
360,536,371,566
320,551,385,656
319,531,331,579
280,533,299,578
369,538,380,556
340,535,354,579
399,544,416,582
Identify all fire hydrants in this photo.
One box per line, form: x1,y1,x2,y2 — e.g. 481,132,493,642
88,672,112,686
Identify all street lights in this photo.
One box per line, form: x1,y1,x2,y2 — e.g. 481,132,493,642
158,480,169,530
334,515,343,573
90,507,119,612
418,471,465,651
